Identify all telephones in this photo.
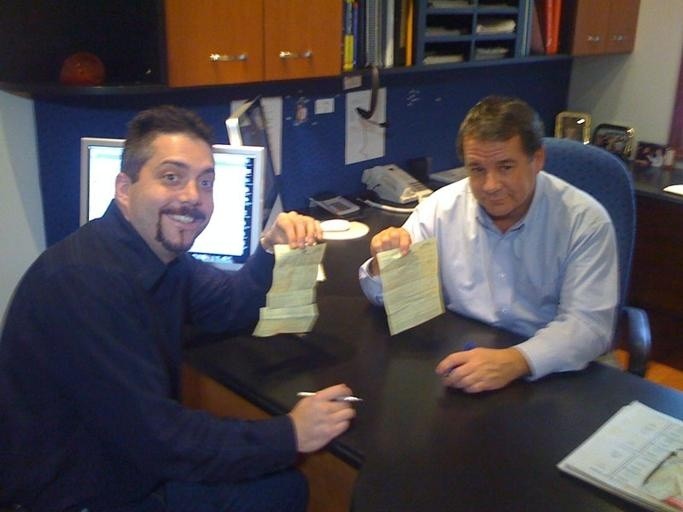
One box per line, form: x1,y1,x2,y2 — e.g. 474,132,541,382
360,163,434,205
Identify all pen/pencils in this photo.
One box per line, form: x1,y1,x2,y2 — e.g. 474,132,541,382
296,392,364,402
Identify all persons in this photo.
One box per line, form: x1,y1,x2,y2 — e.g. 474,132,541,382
358,95,620,395
0,106,356,512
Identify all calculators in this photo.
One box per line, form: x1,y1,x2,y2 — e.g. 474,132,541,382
308,191,360,216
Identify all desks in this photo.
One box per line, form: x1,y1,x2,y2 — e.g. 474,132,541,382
175,160,683,512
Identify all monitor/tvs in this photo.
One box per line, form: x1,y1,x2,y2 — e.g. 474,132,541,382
80,137,267,272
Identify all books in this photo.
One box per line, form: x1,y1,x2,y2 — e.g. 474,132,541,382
555,401,683,512
342,1,414,72
520,0,561,56
423,0,516,65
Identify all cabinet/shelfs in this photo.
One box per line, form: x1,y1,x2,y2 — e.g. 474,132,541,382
416,0,525,65
164,0,343,88
572,0,640,55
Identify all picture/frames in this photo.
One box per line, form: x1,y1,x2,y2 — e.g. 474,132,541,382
554,112,591,145
592,122,633,158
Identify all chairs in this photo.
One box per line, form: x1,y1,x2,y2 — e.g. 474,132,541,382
544,139,650,378
